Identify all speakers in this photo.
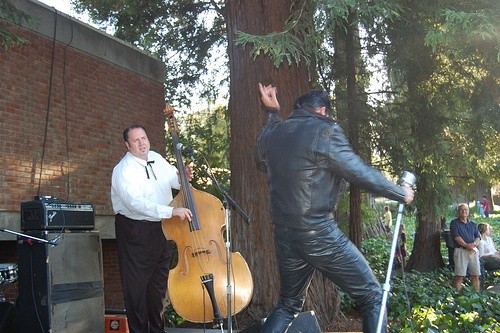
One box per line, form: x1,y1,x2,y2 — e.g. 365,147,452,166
15,231,106,333
239,310,323,333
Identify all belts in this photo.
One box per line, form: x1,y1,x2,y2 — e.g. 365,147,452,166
458,246,466,249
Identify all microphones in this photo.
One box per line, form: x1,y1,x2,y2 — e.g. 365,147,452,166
176,143,199,154
396,171,415,213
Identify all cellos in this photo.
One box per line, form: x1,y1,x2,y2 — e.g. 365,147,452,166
159,101,254,325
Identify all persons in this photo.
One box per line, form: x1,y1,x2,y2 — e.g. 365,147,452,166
254,81,415,333
476,222,500,270
384,205,392,233
394,224,407,268
450,203,482,294
478,196,489,219
108,125,195,333
